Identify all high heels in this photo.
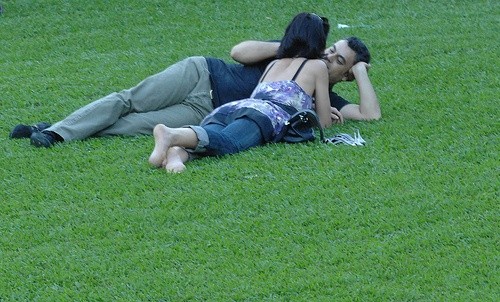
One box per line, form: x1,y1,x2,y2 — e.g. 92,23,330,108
335,129,366,146
325,133,362,148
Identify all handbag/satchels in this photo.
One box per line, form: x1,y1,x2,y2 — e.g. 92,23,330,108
275,110,324,143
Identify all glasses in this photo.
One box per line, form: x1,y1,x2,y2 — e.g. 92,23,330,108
311,12,323,22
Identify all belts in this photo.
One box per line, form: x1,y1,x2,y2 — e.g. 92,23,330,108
209,66,216,110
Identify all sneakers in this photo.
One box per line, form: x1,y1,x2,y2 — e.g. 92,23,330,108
10,122,52,138
30,131,56,147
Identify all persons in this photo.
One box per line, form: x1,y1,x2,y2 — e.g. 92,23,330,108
8,36,382,149
148,12,345,175
